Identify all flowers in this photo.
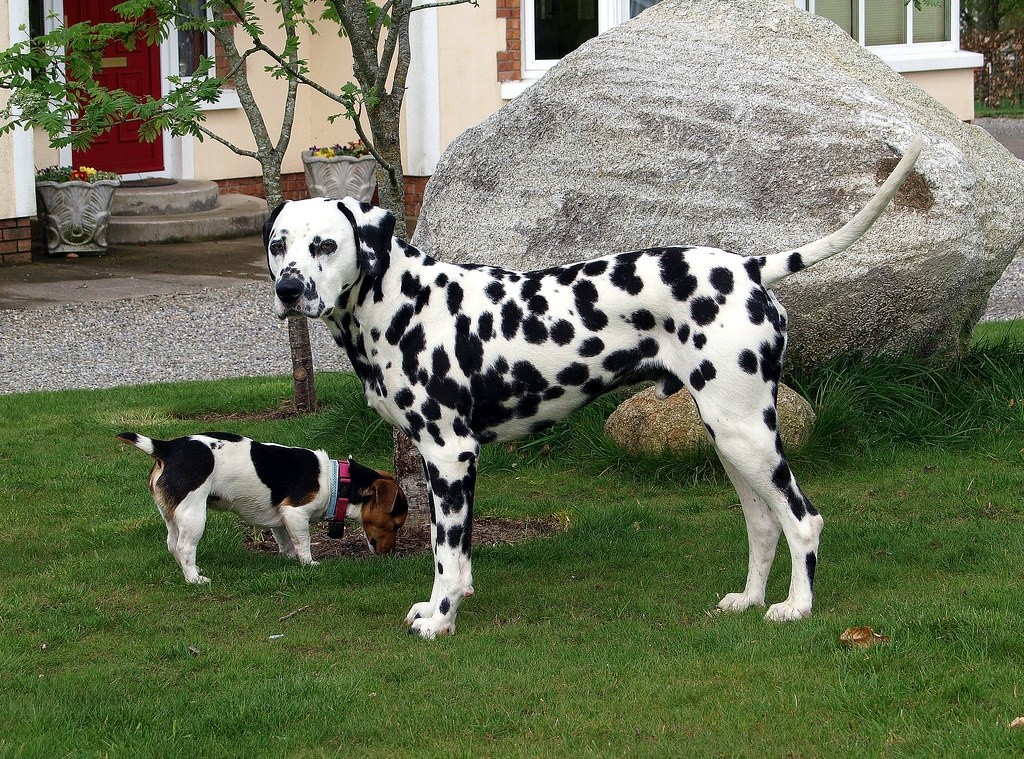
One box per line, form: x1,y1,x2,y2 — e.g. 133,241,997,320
35,162,119,182
309,139,370,159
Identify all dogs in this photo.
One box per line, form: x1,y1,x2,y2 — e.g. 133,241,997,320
114,428,412,587
261,135,932,645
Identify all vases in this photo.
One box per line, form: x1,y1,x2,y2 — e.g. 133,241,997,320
302,150,377,205
35,175,121,255
